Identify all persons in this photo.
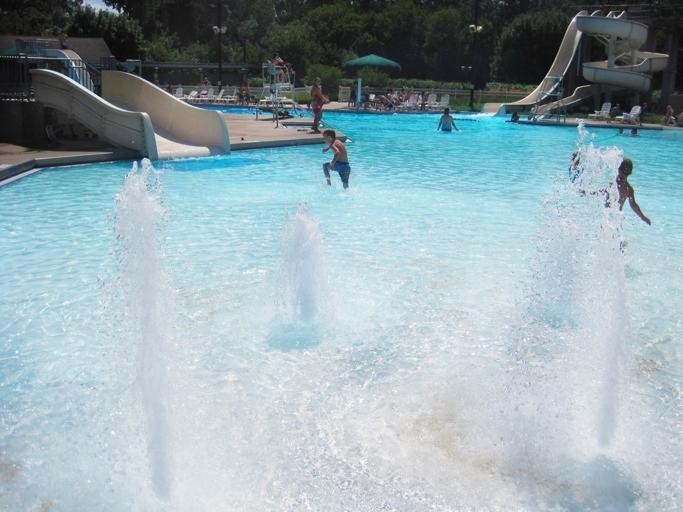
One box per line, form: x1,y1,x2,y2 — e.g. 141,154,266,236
347,81,461,132
666,105,676,126
511,112,520,122
639,103,648,126
311,77,329,134
617,127,639,136
569,153,580,184
578,157,651,225
322,129,351,189
238,81,251,106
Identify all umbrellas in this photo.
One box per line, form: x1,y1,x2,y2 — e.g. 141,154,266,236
345,54,402,94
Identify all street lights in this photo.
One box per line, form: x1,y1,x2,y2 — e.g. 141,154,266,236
212,0,228,92
469,0,483,106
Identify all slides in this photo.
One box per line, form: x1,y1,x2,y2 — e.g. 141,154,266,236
483,9,601,117
28,68,231,163
531,11,669,113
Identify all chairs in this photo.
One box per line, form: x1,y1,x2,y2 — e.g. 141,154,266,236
587,101,643,124
361,93,450,112
172,88,238,106
